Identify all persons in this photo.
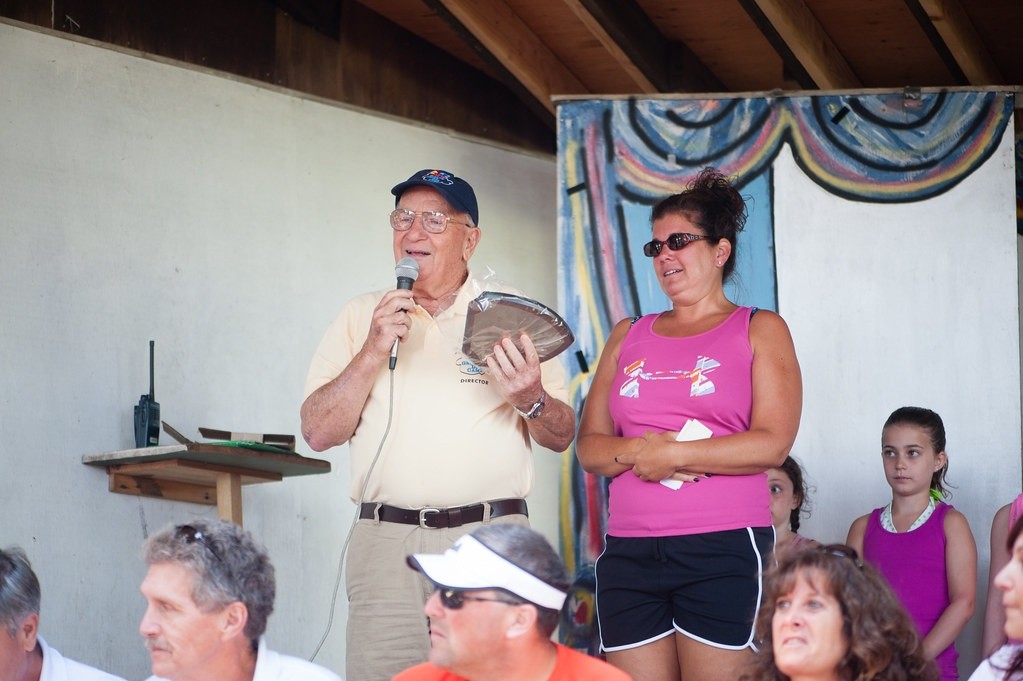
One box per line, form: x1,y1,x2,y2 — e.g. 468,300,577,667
979,493,1023,665
764,455,822,561
738,542,941,681
577,165,803,679
298,169,577,681
389,522,634,681
967,512,1023,681
845,406,978,681
135,517,343,681
0,546,126,680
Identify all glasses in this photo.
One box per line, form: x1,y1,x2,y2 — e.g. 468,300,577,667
817,544,864,571
433,586,520,610
390,209,473,234
643,233,716,257
174,525,221,562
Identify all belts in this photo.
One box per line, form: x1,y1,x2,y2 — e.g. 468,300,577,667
358,498,528,531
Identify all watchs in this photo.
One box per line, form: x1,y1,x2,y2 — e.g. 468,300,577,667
511,389,549,423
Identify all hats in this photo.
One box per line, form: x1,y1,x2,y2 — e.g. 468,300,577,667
391,169,479,228
405,533,568,613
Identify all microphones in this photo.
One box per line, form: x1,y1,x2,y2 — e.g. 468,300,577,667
389,256,419,370
